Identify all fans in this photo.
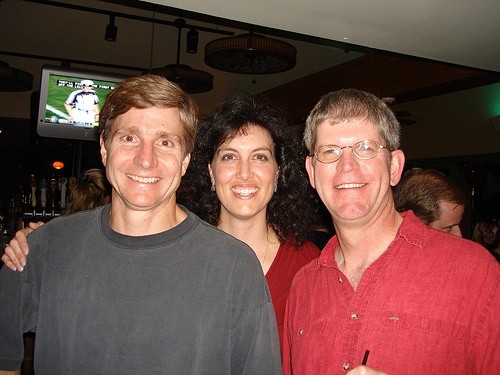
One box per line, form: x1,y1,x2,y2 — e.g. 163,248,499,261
139,18,214,95
204,26,297,86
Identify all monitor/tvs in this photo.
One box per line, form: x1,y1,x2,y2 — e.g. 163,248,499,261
37,65,135,140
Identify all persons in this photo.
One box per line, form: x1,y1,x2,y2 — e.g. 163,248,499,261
283,89,500,375
2,94,323,375
63,81,100,124
0,74,285,375
60,170,500,260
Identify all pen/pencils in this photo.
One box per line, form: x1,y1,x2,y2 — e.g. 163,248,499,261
361,350,370,366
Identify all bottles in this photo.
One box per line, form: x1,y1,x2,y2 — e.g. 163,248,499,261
39,178,50,207
30,174,38,208
49,172,59,208
9,198,15,208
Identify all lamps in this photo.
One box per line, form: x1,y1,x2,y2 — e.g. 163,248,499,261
185,27,200,54
105,15,118,41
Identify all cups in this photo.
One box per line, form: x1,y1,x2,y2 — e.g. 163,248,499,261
20,191,31,206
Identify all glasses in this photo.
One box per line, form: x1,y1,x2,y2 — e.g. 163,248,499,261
309,140,390,164
